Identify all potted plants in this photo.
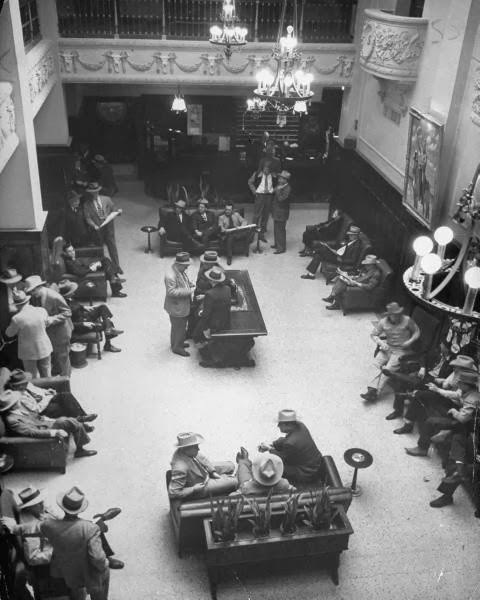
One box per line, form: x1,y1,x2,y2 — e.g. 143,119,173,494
207,484,339,544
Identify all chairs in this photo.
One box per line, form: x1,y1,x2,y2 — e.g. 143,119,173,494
373,306,479,503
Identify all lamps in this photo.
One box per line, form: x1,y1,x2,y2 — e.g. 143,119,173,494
208,1,250,62
252,1,317,129
401,163,480,323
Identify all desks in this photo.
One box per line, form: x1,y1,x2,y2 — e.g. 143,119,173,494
209,269,269,370
200,503,355,600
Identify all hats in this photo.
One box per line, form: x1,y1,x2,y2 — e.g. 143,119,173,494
277,410,298,422
252,454,284,486
0,269,77,306
17,487,46,510
176,432,204,447
65,190,82,202
175,200,185,208
56,486,88,515
91,154,108,165
362,255,380,265
384,303,404,314
449,355,478,385
346,226,361,234
1,368,32,411
86,183,103,193
176,251,226,282
278,170,291,179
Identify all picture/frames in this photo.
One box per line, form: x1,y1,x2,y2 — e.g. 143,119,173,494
399,105,447,235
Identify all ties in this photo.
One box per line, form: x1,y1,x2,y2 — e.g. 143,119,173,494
95,199,104,218
265,175,268,193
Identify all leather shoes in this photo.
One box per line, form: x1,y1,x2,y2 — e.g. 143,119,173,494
407,447,428,456
382,369,396,378
431,429,452,443
386,411,401,419
301,273,315,279
107,557,124,569
442,472,470,484
104,328,124,352
236,447,248,463
93,508,121,524
113,268,126,297
323,295,340,309
260,236,284,254
430,494,453,507
395,422,413,434
183,330,204,355
75,412,97,457
360,392,377,400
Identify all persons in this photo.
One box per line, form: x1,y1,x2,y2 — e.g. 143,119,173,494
3,368,98,457
158,199,248,265
270,171,291,254
299,208,380,309
163,251,237,356
62,154,127,297
248,133,282,243
386,336,478,518
168,409,323,505
361,302,420,401
1,486,125,598
3,269,124,376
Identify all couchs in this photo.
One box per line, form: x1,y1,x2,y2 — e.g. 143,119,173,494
158,205,251,260
164,454,355,559
0,242,109,599
304,208,395,318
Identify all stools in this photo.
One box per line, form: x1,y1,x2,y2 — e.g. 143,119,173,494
140,226,158,254
